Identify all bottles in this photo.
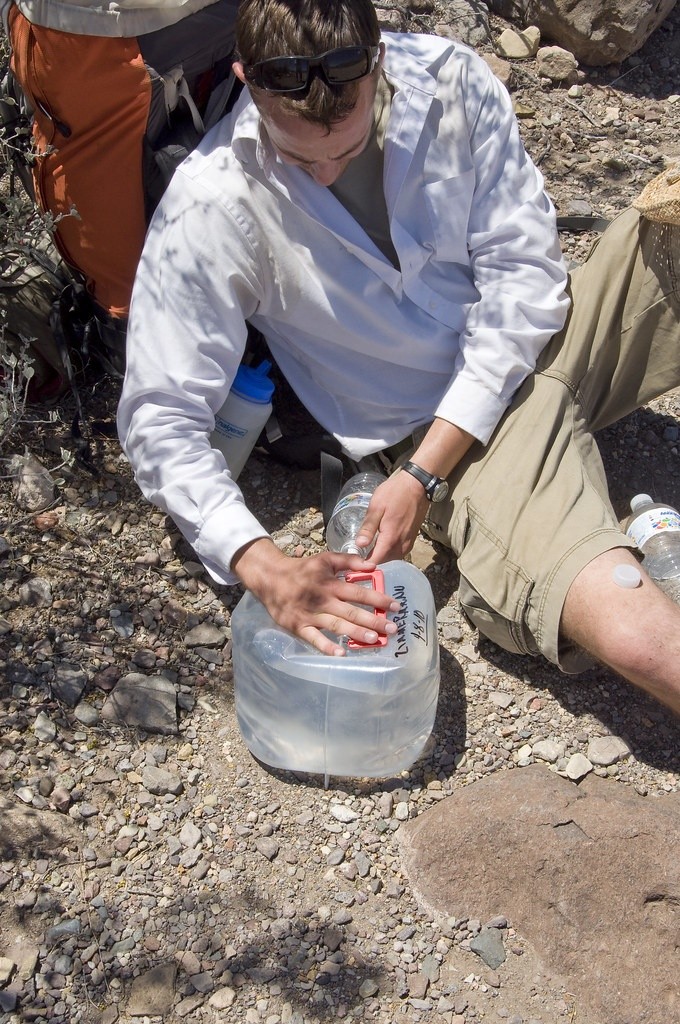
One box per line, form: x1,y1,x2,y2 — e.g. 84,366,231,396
209,358,275,484
325,468,388,564
625,491,680,608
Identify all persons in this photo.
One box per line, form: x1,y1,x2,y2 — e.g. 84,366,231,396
115,0,680,718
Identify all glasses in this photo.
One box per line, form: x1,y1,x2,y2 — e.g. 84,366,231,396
244,44,380,92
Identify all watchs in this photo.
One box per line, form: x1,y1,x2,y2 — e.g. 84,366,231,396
401,461,450,504
